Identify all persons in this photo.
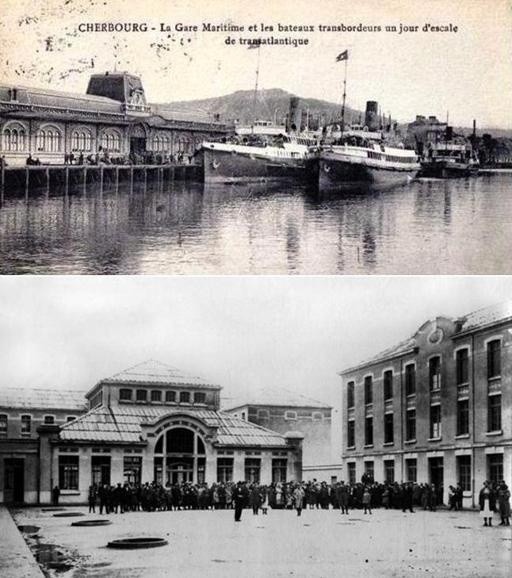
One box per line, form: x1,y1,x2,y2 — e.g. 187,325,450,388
2,155,8,166
35,157,41,165
26,155,34,164
88,472,509,527
428,148,433,161
64,150,185,167
53,485,60,505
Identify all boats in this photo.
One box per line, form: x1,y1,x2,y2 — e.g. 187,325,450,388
191,49,480,196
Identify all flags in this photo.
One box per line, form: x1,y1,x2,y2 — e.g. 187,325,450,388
335,50,347,62
247,40,260,49
